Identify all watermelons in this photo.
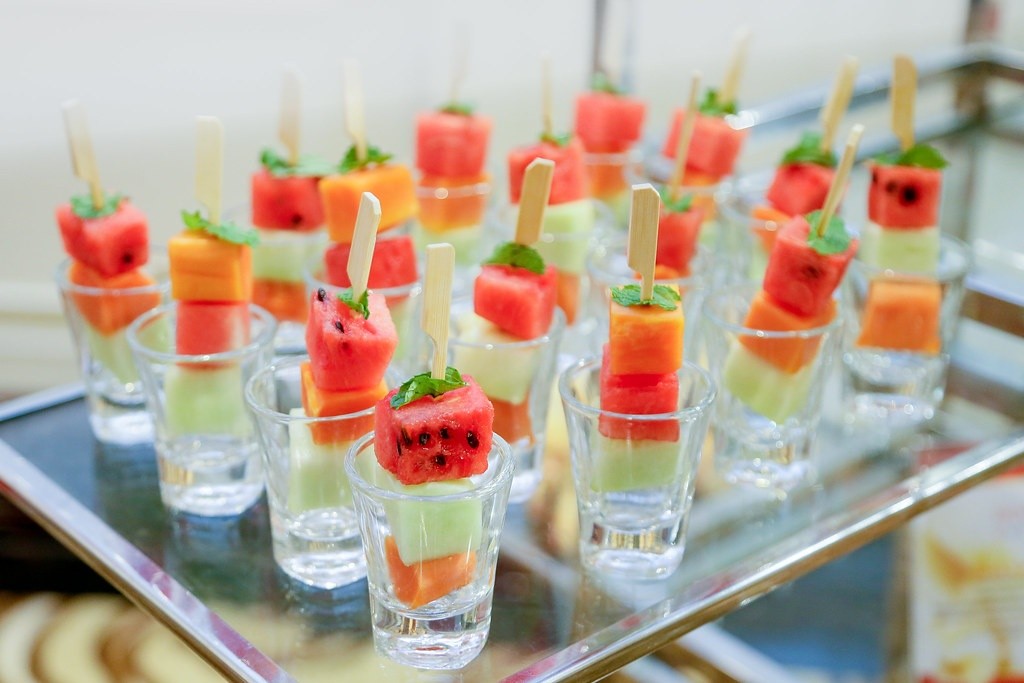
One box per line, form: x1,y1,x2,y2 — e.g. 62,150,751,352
55,99,938,483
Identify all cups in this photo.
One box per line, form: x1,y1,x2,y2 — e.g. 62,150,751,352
124,304,277,516
56,251,178,444
558,355,717,581
344,431,513,669
244,362,399,589
232,143,970,503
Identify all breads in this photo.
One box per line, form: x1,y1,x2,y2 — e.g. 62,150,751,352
0,589,526,683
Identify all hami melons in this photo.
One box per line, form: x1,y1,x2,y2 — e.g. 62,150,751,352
76,164,935,611
